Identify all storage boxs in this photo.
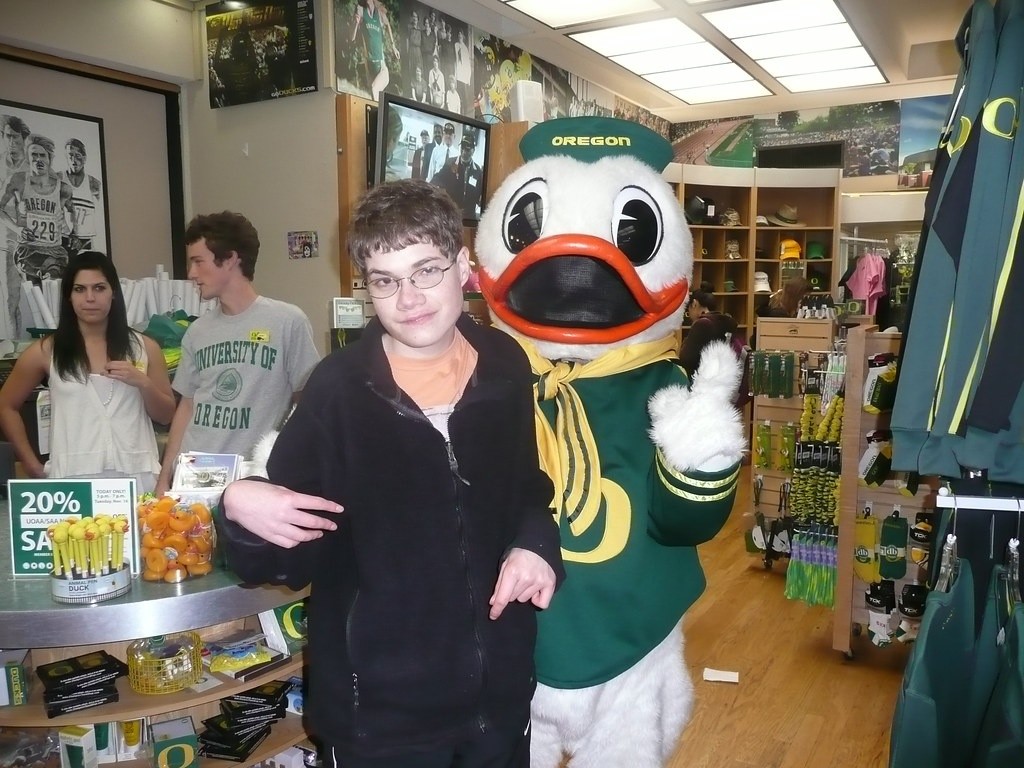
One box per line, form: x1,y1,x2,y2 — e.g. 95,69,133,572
0,600,308,768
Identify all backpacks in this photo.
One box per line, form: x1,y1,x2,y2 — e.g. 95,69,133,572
697,314,753,408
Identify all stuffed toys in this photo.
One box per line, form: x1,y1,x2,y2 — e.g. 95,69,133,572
250,116,751,768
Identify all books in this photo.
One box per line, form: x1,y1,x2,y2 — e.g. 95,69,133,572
35,649,129,719
257,597,308,657
196,678,296,763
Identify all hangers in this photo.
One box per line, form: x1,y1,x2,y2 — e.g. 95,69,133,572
934,491,1024,604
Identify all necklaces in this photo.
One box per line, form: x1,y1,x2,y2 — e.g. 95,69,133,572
90,376,114,406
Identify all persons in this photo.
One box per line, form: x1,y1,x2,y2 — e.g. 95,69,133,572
349,0,400,102
568,96,601,118
208,11,294,109
757,126,899,176
385,105,484,222
678,281,737,392
404,8,472,117
0,114,103,340
216,177,566,768
670,117,741,160
0,250,175,498
152,210,320,499
754,276,813,318
613,105,670,141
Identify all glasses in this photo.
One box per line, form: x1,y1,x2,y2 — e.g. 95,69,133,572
685,311,690,317
361,257,457,299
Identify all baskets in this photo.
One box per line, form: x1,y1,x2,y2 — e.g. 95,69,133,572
127,630,201,695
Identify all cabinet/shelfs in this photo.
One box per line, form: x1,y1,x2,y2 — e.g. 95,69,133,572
661,161,843,358
0,500,316,768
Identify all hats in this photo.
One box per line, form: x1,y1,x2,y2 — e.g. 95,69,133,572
462,133,475,148
754,272,772,292
447,123,455,135
684,194,716,224
767,204,807,227
725,279,738,292
756,215,769,225
726,240,742,260
780,239,801,260
808,270,829,292
806,241,826,259
718,207,742,226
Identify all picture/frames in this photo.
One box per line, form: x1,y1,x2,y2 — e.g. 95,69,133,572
0,100,114,363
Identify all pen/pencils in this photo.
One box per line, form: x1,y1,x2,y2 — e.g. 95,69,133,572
46,514,130,581
